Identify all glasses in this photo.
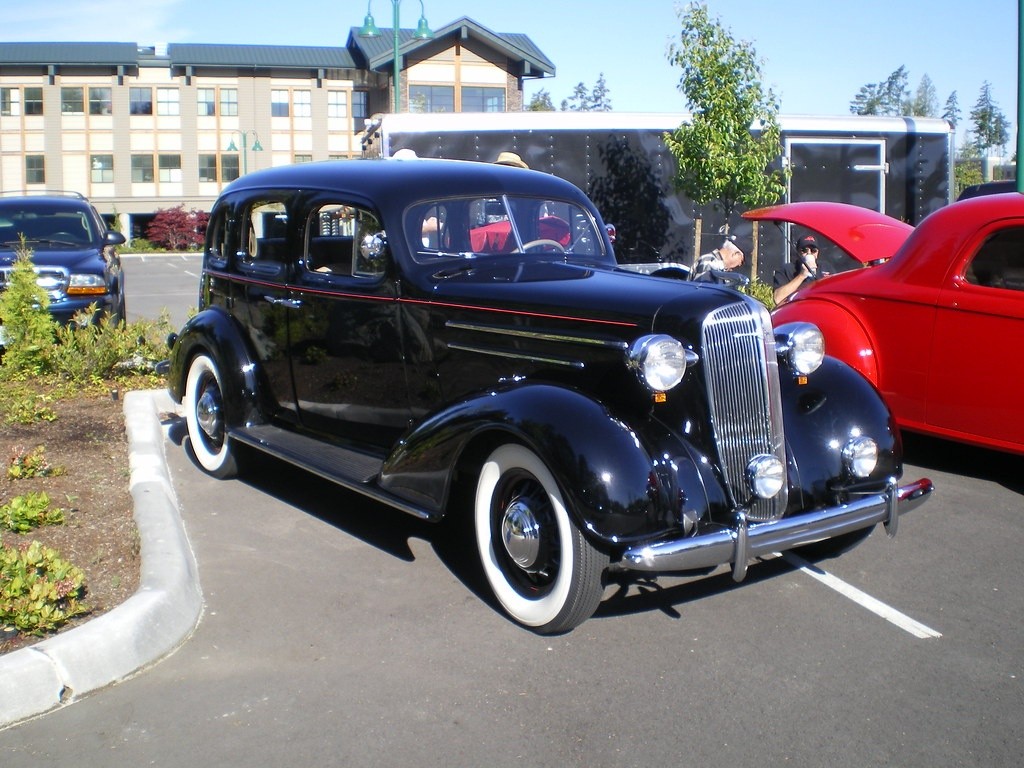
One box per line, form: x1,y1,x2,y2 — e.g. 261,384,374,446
801,246,818,253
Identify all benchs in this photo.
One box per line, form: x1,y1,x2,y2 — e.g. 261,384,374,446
255,236,353,268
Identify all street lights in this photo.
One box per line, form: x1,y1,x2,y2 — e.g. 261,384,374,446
357,0,437,114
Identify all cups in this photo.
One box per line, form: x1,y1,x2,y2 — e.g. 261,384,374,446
805,255,816,277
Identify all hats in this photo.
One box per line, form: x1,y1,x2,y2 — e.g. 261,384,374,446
727,232,752,261
796,234,820,249
493,152,529,169
392,149,418,159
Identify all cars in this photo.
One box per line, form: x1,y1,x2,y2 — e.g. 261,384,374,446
157,157,934,636
0,189,127,331
740,191,1024,457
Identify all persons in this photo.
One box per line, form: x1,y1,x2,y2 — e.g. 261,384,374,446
477,151,547,224
772,234,830,306
392,149,437,248
686,232,754,282
